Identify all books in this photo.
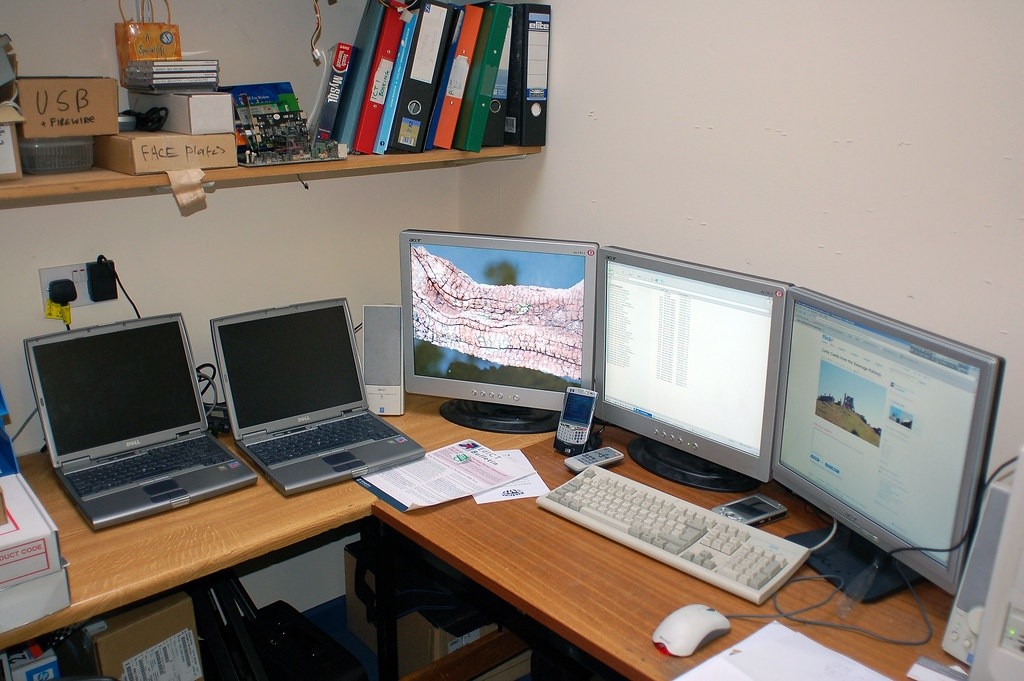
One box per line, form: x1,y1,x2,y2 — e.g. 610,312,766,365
310,0,512,153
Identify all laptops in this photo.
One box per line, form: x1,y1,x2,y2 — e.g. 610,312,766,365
23,313,258,533
210,297,427,496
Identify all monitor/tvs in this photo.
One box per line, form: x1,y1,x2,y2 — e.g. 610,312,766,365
400,229,599,435
592,246,796,493
772,286,1005,603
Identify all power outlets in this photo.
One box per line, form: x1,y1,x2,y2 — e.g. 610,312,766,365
39,263,119,311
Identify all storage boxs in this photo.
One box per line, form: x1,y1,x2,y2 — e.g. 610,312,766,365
80,590,206,681
14,75,119,138
18,139,94,174
0,557,72,635
0,474,62,590
0,105,25,181
344,544,499,674
127,88,233,135
92,129,238,175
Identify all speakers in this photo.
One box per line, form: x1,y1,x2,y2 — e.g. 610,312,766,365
361,305,405,415
943,482,1012,666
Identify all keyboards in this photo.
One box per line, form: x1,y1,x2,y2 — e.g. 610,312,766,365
535,464,810,605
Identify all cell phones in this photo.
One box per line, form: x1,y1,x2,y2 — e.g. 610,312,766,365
553,386,598,457
710,492,789,529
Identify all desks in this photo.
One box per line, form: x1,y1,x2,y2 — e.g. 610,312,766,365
371,426,972,681
1,393,557,653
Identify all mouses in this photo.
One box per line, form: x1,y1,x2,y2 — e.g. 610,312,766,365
651,604,731,657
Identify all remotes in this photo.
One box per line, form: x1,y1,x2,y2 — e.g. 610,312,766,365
565,447,624,475
